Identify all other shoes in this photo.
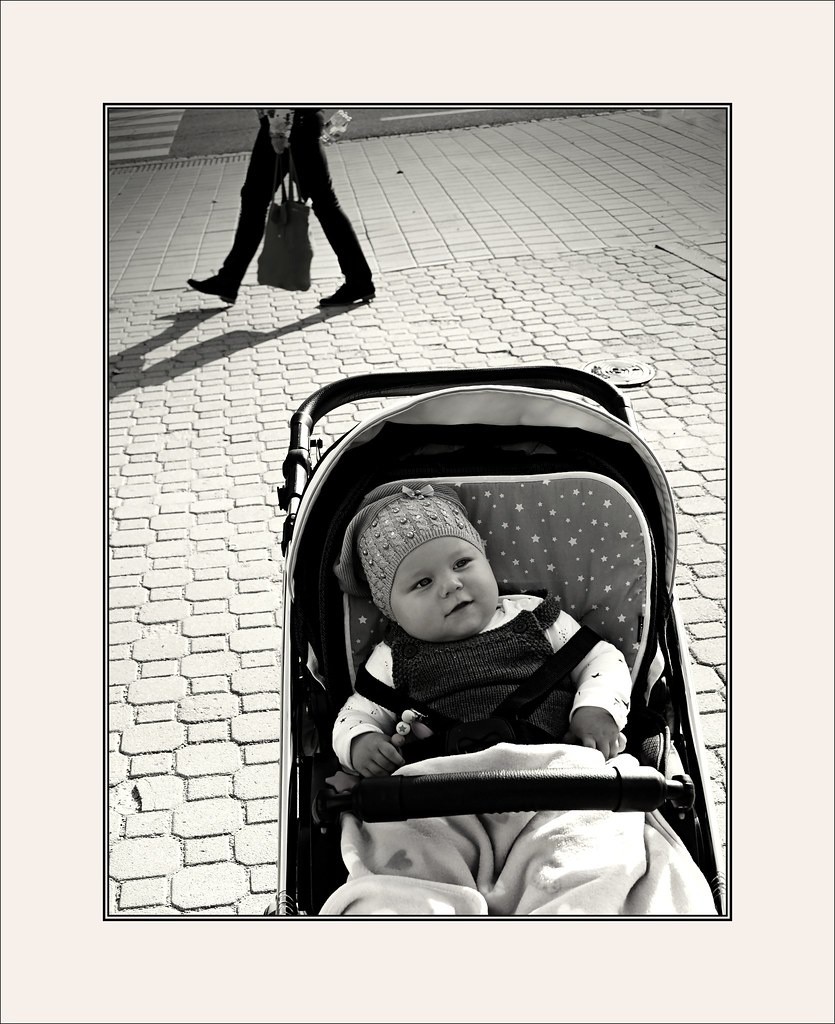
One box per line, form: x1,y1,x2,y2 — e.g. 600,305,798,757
321,280,377,307
187,264,242,304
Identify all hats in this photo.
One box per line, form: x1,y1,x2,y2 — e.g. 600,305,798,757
359,485,486,628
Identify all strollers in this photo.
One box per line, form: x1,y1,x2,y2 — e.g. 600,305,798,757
277,363,727,920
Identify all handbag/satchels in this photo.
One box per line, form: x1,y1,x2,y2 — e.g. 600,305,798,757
259,145,314,292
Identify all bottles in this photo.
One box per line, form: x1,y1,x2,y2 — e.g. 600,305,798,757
318,109,353,148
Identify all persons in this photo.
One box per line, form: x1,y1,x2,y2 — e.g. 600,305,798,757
186,108,375,307
317,483,719,915
